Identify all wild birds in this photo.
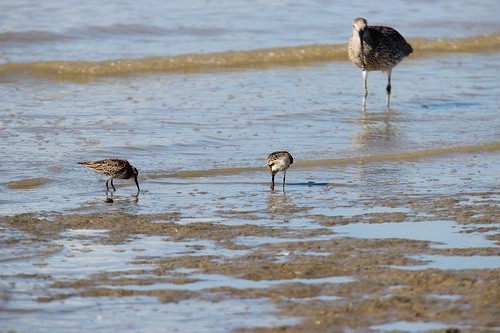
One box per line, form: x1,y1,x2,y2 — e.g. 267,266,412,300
347,18,414,110
77,158,141,194
266,151,294,193
